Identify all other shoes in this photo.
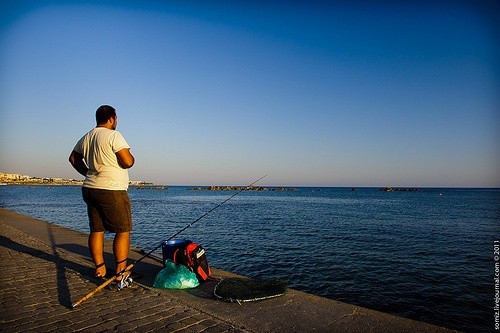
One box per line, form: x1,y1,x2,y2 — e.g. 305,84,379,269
116,275,132,289
94,275,107,287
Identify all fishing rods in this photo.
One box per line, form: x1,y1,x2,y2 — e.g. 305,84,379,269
71,174,269,308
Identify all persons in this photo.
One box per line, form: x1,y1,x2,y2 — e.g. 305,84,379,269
68,105,144,285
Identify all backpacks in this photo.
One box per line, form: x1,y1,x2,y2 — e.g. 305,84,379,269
163,240,212,283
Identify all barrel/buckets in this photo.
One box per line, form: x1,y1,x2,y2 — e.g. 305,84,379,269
162,239,192,269
